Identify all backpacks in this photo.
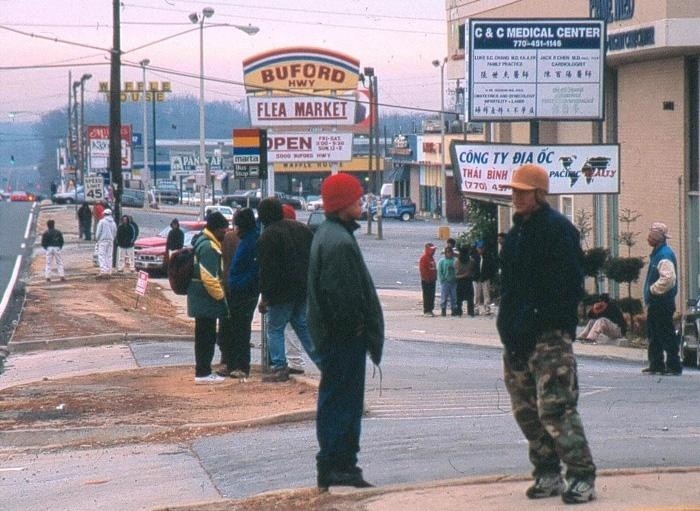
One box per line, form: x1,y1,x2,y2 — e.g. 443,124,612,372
166,248,194,294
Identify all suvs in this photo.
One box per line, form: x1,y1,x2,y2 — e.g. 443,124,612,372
155,179,179,203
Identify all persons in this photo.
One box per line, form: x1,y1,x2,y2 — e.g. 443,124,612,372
163,218,185,260
41,220,67,282
189,197,323,384
575,301,627,344
305,173,384,489
77,197,139,279
418,232,510,317
496,164,596,504
50,180,56,204
640,221,684,376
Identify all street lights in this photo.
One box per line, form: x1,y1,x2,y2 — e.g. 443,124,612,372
111,21,263,213
184,7,215,220
431,56,448,225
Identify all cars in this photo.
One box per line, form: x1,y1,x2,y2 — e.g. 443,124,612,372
10,190,30,201
180,188,417,222
52,185,108,204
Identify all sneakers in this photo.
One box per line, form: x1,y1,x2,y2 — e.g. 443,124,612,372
424,307,491,316
262,369,304,382
562,477,596,503
526,477,565,499
217,363,249,378
196,375,224,384
574,337,596,345
641,367,682,376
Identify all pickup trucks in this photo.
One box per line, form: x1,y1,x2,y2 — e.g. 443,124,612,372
134,220,209,255
203,205,235,231
136,231,201,272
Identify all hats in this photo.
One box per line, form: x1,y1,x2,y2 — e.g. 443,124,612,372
208,212,228,229
648,222,673,241
102,209,112,214
282,204,296,219
321,173,364,213
499,164,549,194
